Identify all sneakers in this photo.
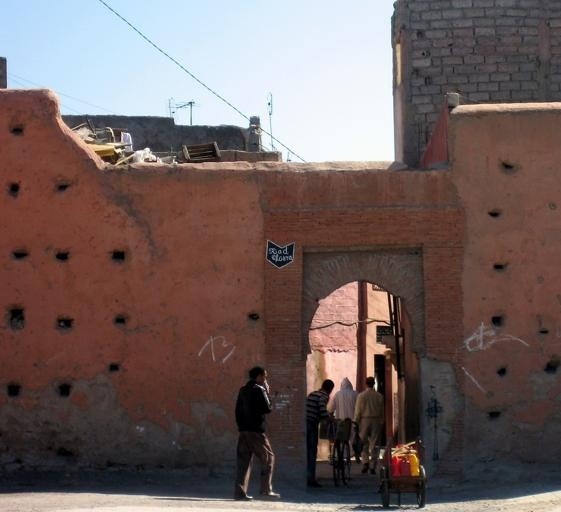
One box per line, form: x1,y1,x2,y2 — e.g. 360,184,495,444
362,464,376,474
308,480,323,487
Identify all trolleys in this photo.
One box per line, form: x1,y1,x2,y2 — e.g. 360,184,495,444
377,437,427,508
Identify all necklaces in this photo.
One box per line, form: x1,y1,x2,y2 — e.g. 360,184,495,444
306,378,337,488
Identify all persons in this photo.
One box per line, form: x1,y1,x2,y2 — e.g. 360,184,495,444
234,366,285,502
352,376,387,474
329,376,361,458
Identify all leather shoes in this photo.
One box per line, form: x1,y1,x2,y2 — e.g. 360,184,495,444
235,495,253,500
260,491,280,498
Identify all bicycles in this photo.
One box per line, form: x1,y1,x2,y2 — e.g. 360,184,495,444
329,418,359,488
